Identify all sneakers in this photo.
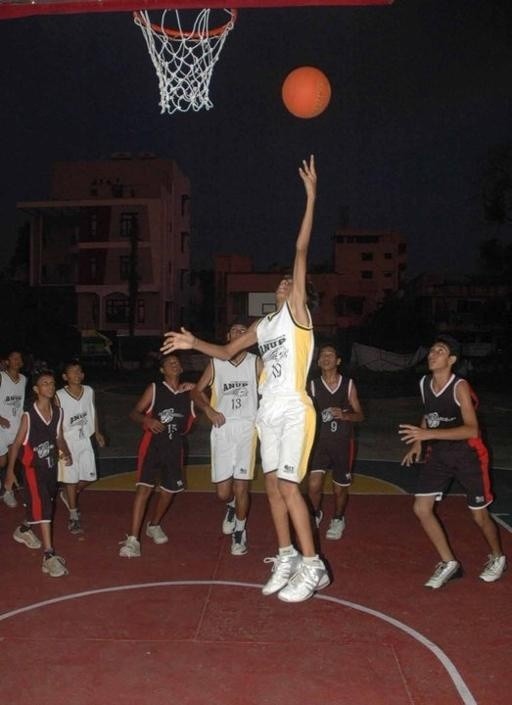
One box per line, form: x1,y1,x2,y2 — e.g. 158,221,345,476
425,561,460,589
13,525,42,549
262,551,303,595
68,519,84,535
60,489,72,512
230,530,248,555
146,521,169,544
326,516,345,540
278,559,330,602
312,510,323,529
119,536,141,557
222,505,236,534
42,554,69,577
4,491,17,508
478,553,507,582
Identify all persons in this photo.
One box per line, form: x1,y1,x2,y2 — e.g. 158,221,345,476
0,350,29,510
189,321,265,558
3,371,74,578
158,152,333,607
53,360,107,537
395,336,511,593
306,343,365,543
117,352,210,560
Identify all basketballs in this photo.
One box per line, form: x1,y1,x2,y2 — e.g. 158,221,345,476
283,67,330,119
